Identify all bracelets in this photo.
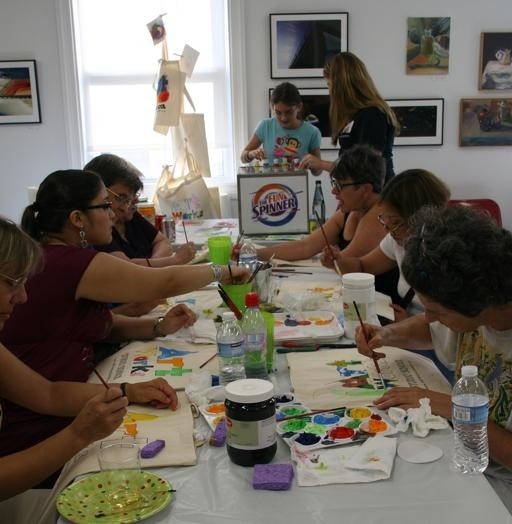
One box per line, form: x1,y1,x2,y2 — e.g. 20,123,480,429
208,264,223,282
119,381,129,405
246,150,254,160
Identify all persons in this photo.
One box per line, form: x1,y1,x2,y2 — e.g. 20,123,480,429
93,175,198,268
294,50,398,210
81,153,168,363
355,200,512,516
0,168,253,458
239,81,323,177
231,142,389,264
318,168,452,325
0,214,179,524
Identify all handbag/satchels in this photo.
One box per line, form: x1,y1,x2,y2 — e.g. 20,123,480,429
152,166,221,220
154,61,186,135
171,114,212,177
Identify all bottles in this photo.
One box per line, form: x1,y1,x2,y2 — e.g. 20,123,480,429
138,197,148,203
239,292,268,380
342,272,375,339
216,311,246,386
309,215,317,234
238,238,257,272
255,158,299,175
313,180,325,226
451,365,489,475
135,202,156,227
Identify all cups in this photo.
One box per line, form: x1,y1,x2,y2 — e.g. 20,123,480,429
97,443,141,511
221,279,253,312
244,311,275,372
239,261,273,303
208,236,232,266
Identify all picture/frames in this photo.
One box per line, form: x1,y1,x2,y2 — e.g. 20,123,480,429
269,87,342,151
458,99,512,147
384,98,444,146
478,32,512,91
269,12,349,79
0,60,41,124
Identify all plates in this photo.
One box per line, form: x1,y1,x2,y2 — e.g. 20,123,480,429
55,470,173,524
270,311,344,345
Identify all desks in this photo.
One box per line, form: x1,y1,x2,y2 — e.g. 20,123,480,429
36,219,511,524
136,202,155,217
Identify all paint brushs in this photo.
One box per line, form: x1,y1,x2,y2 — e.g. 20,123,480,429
352,300,387,391
273,269,295,271
285,271,312,274
93,368,109,390
145,256,152,267
283,341,357,348
217,284,242,321
182,222,188,243
237,230,244,248
200,353,217,368
228,265,233,284
315,210,343,277
248,264,263,283
276,407,346,421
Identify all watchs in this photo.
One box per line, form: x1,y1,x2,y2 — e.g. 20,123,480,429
154,316,167,337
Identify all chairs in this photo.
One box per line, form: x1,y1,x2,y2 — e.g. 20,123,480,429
443,200,502,231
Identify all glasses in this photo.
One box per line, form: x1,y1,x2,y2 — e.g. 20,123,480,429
377,214,404,235
0,273,27,286
106,188,132,207
88,201,113,210
378,215,384,225
331,180,353,192
